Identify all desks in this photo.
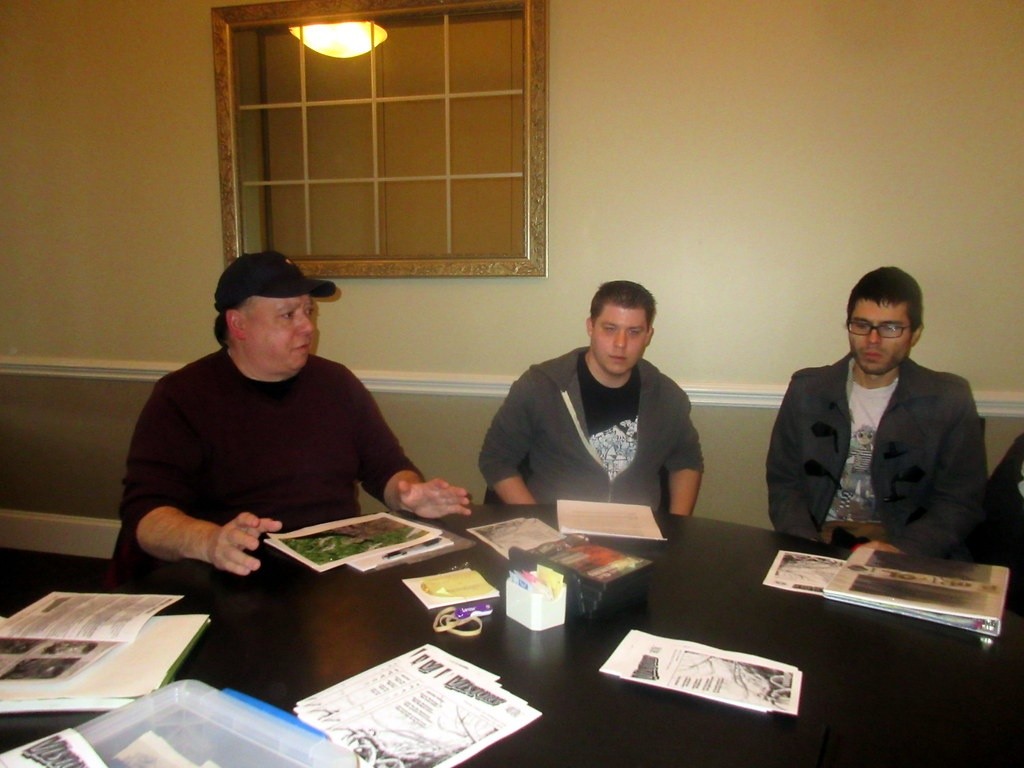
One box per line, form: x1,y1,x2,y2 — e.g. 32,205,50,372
0,504,1024,768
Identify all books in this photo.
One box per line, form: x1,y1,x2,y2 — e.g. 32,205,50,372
0,591,212,713
820,544,1009,637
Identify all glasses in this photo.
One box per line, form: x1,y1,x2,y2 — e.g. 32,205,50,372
846,319,910,338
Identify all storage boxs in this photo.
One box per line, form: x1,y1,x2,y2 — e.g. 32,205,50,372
72,680,361,768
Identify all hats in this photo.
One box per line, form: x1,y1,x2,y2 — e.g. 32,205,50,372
214,251,337,308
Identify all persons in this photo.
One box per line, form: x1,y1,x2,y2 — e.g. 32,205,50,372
765,268,987,556
477,280,703,518
117,251,472,575
